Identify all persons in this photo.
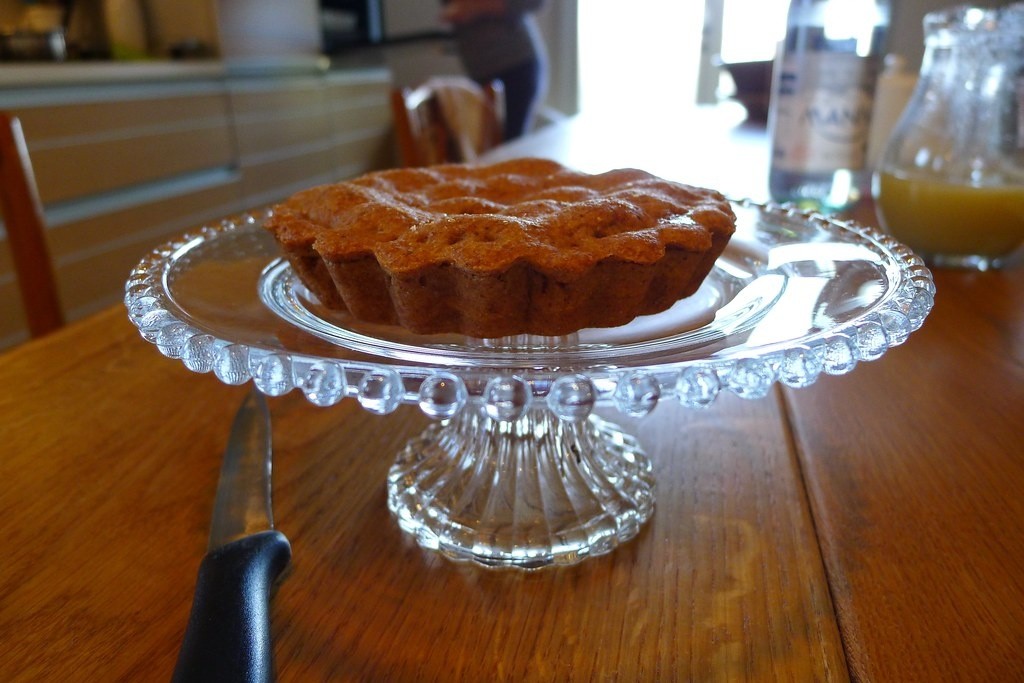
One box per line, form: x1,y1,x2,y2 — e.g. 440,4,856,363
435,0,548,144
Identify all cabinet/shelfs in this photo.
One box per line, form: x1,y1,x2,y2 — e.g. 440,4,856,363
3,66,394,352
387,78,508,171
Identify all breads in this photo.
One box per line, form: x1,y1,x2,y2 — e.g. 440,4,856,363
265,159,738,341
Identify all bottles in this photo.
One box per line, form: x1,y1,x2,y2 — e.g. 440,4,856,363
767,0,892,216
870,7,1024,270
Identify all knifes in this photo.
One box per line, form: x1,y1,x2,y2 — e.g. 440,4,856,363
169,383,291,683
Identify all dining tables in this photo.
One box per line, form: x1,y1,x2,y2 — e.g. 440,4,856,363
0,105,1024,683
